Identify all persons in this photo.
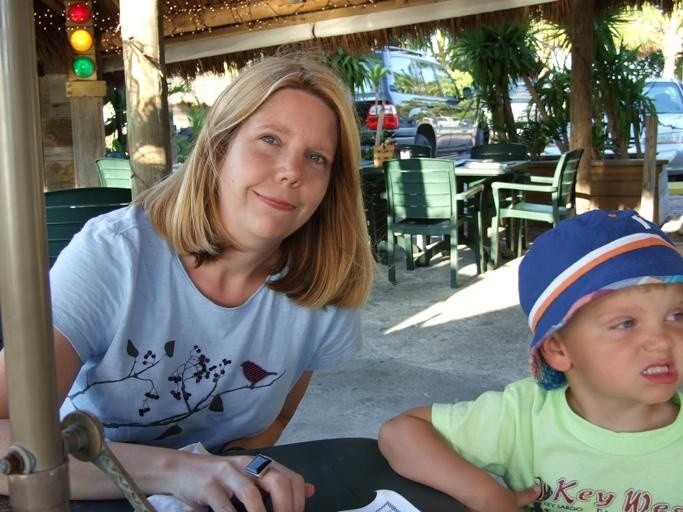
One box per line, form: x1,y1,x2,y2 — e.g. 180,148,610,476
2,55,370,511
379,209,683,512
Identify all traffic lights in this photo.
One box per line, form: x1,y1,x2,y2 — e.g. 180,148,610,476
63,0,98,80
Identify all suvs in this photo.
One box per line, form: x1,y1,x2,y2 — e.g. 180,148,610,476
354,46,490,159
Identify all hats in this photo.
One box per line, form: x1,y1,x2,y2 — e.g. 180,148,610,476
518,209,682,390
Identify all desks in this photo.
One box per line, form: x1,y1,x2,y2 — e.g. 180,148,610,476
72,437,474,512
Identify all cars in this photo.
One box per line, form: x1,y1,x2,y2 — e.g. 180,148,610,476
484,81,683,176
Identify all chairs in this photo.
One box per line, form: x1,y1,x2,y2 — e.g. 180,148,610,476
358,143,585,288
44,188,131,267
94,158,135,188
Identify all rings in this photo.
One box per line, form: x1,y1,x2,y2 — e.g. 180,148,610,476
245,454,274,484
